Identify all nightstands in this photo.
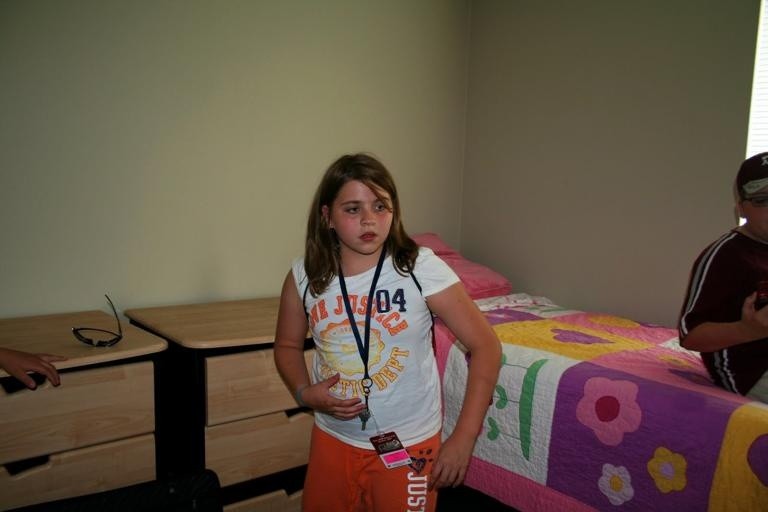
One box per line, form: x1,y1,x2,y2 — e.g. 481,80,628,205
1,309,168,511
123,296,317,512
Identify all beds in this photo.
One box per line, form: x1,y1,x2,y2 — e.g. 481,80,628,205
428,291,767,511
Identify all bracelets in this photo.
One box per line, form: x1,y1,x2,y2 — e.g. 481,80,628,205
297,384,308,408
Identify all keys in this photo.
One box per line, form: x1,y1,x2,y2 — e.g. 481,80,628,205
357,407,372,431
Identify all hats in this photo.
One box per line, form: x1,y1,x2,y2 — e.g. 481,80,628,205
736,151,768,198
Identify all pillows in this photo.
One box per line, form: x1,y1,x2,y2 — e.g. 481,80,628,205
408,232,511,300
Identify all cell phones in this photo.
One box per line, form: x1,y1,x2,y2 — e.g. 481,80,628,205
757,281,768,311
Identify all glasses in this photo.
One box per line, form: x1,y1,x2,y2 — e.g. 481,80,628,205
741,195,768,206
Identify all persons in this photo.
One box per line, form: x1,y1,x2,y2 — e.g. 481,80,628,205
0,346,67,391
680,152,768,403
273,152,503,512
